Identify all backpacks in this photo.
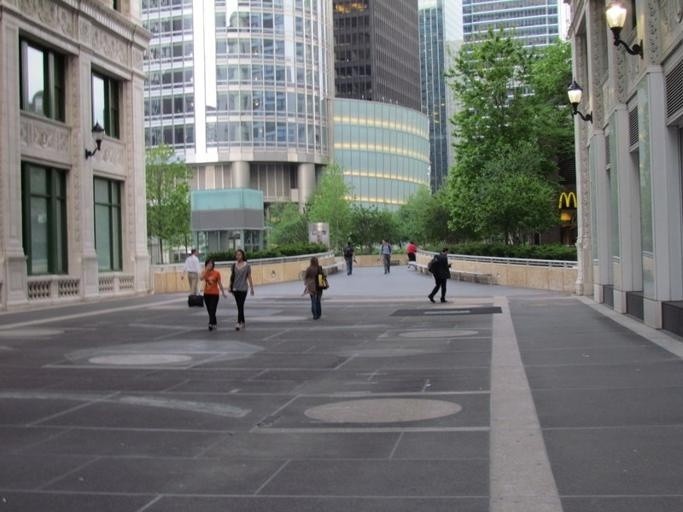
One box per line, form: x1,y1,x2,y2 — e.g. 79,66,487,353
428,255,441,274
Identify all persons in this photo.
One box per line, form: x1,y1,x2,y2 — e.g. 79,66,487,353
229,248,254,330
199,257,228,330
304,256,330,320
180,248,202,295
406,239,416,261
343,240,354,276
428,247,454,303
376,238,391,273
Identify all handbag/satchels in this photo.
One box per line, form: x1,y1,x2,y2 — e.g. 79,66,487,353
315,266,329,290
188,295,204,308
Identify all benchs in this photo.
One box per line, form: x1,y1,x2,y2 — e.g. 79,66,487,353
407,261,492,284
316,261,344,274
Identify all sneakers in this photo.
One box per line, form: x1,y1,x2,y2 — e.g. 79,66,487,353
440,297,447,303
313,314,321,320
428,294,435,302
347,271,352,276
236,321,246,331
385,269,390,274
209,324,217,331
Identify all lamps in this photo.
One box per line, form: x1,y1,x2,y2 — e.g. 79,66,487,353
85,121,105,159
565,78,593,127
604,0,644,62
